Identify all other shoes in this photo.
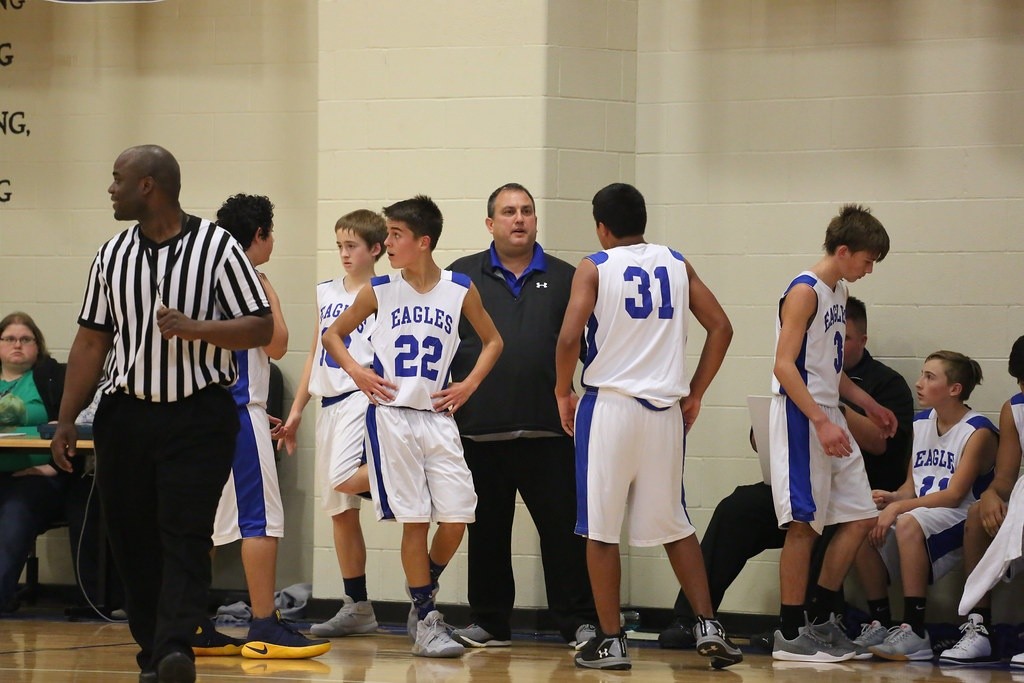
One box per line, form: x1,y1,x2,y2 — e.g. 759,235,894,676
139,669,158,683
111,609,128,619
158,652,197,683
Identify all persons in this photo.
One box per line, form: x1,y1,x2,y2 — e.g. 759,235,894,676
772,200,899,662
554,183,743,670
322,193,503,658
0,310,85,618
68,388,127,621
939,335,1024,669
659,295,914,654
49,143,274,683
812,349,998,663
443,183,597,648
270,206,388,637
189,191,331,659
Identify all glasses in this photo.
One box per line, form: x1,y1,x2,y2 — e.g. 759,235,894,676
0,335,36,345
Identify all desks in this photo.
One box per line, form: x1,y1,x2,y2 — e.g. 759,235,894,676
0,423,116,619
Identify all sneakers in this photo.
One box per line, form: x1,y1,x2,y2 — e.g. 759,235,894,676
240,651,331,678
940,666,992,683
852,620,890,646
691,614,743,669
804,608,874,660
411,612,465,658
939,613,1004,665
1010,652,1024,669
868,623,933,661
193,619,247,657
451,621,511,648
573,626,631,671
310,593,377,636
404,573,439,642
658,621,694,649
772,658,855,676
568,622,597,650
748,622,782,650
242,609,330,659
194,651,241,669
772,626,857,663
1010,672,1024,682
